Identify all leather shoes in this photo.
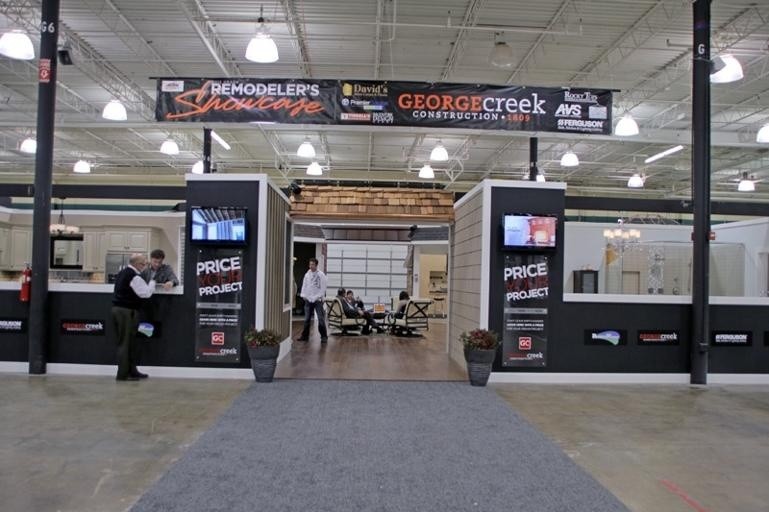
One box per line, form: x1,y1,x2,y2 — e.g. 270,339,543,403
296,337,307,342
321,338,327,343
114,377,138,382
130,372,148,379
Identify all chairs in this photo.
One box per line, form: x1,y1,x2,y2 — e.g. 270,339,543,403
433,290,446,318
323,294,433,338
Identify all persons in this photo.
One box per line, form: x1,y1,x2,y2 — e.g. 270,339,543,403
110,252,148,381
140,249,178,290
391,291,413,336
297,257,329,344
335,287,385,335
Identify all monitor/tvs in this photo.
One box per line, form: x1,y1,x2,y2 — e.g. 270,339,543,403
499,211,559,253
187,205,249,248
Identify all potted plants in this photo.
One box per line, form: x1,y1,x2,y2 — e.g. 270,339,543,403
458,328,501,387
245,328,282,382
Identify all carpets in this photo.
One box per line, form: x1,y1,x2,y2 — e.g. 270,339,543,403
126,379,631,512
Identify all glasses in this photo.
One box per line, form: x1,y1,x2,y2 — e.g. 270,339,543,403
136,262,145,267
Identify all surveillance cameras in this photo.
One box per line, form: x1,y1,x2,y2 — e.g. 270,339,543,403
410,225,418,231
